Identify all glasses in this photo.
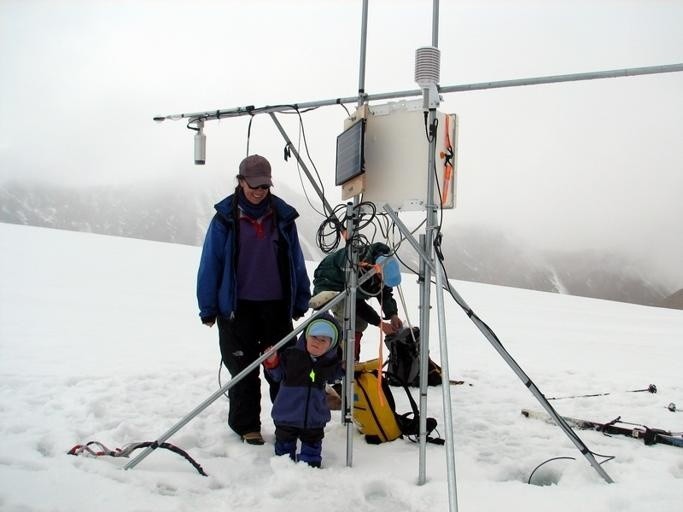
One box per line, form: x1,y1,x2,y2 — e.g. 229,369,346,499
243,178,270,189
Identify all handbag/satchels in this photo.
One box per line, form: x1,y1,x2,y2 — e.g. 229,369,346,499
384,327,420,387
353,370,419,445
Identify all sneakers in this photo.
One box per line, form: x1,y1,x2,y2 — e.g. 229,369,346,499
246,431,264,445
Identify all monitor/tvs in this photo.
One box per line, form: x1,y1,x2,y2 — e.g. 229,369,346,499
335,118,366,186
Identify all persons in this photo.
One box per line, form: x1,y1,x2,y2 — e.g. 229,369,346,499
197,155,311,445
313,243,403,397
263,314,345,467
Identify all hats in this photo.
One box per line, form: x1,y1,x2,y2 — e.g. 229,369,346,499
309,322,333,337
240,154,271,187
376,254,401,287
